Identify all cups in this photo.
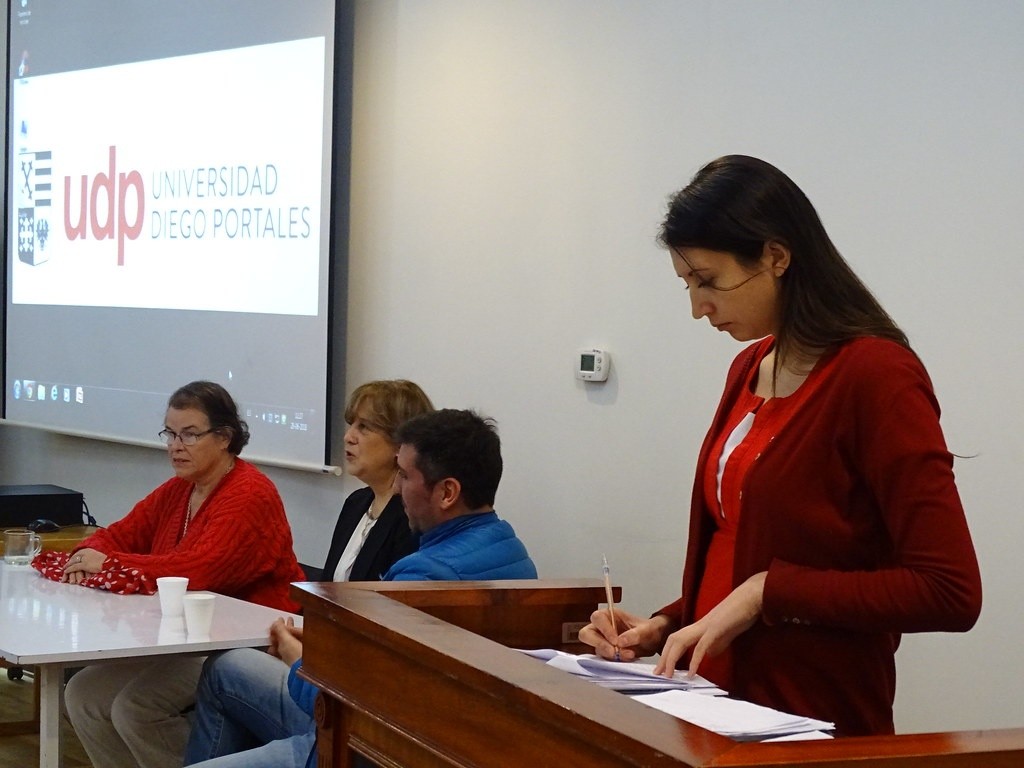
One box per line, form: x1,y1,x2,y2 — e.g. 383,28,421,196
182,593,217,633
3,529,43,565
156,577,189,616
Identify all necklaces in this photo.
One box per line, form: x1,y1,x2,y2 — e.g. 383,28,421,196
345,501,379,575
183,460,234,538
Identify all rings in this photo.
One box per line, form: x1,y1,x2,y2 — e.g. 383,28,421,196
77,556,81,562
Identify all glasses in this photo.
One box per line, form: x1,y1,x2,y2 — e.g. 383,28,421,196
158,429,213,446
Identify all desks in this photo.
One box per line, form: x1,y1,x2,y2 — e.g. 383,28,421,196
0,554,303,768
284,576,1024,768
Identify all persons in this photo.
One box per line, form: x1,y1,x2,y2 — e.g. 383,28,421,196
579,154,982,741
61,381,308,768
182,380,537,768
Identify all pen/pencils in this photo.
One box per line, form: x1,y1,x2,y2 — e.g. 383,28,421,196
603,552,625,662
